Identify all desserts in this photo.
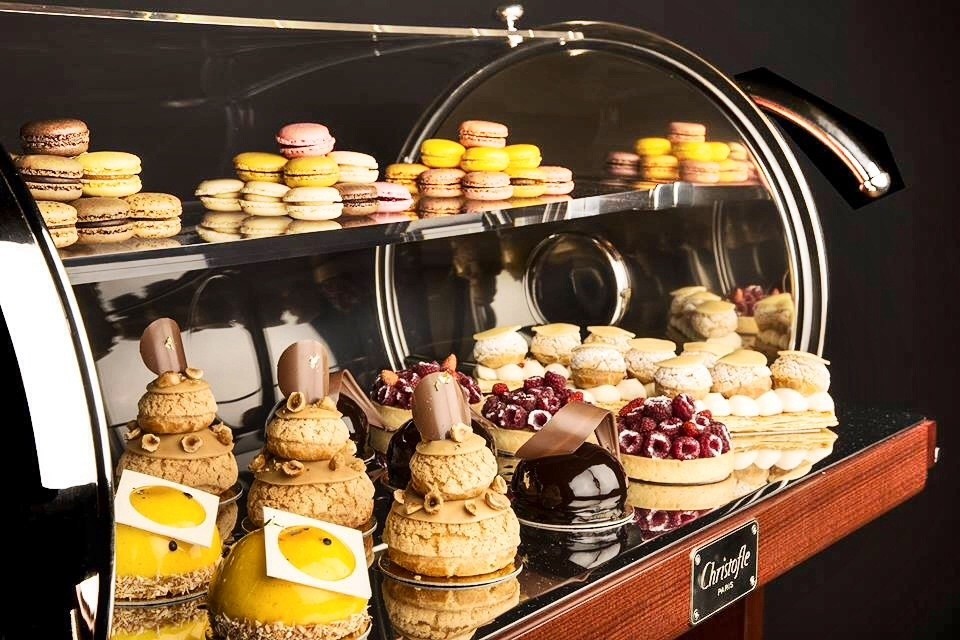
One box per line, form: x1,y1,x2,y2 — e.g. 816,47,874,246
604,121,751,183
111,317,841,640
9,116,575,247
665,285,796,348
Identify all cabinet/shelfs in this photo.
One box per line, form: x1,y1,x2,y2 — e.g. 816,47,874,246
1,1,941,640
